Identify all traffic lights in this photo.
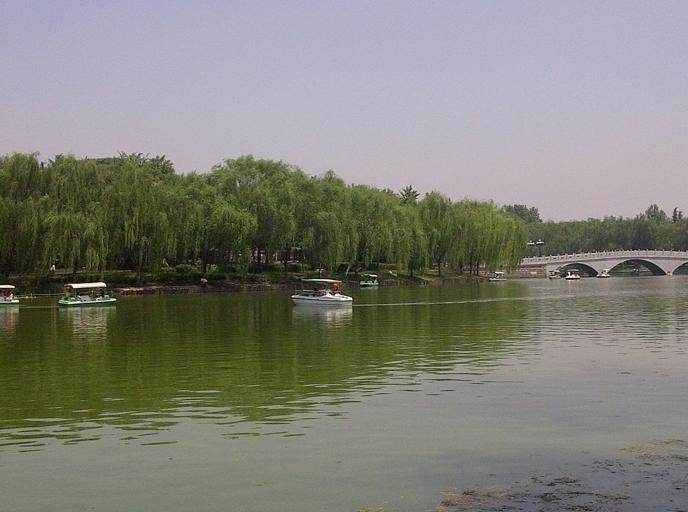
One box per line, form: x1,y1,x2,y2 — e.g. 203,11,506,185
631,266,639,275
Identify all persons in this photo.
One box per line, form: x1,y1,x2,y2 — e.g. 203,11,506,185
96,288,103,300
77,293,82,302
50,264,55,273
6,291,13,301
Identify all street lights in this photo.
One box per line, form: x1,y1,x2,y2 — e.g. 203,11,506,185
527,240,535,257
536,238,544,257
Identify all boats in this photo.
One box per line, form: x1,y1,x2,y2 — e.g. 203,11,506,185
565,269,580,280
0,285,20,304
596,269,610,277
58,281,117,305
360,273,379,286
291,278,353,303
548,270,561,279
489,271,507,281
